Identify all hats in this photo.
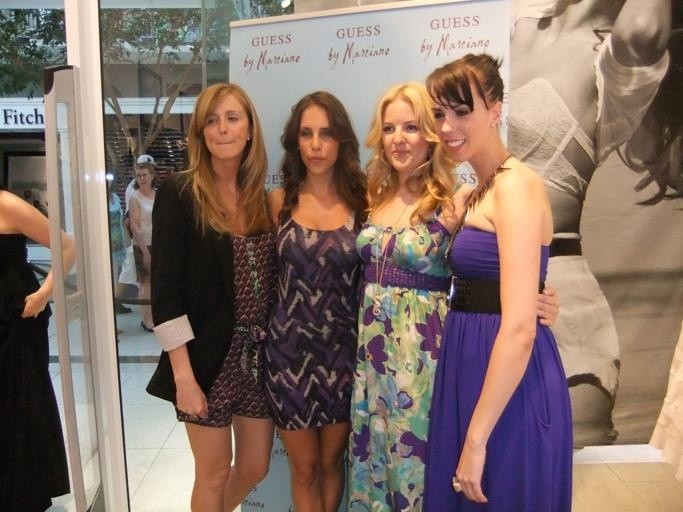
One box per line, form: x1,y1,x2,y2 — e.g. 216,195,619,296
137,154,156,165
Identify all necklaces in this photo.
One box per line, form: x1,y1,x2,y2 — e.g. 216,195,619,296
373,182,419,317
442,152,516,266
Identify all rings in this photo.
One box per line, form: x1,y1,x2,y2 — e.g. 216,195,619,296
450,475,464,493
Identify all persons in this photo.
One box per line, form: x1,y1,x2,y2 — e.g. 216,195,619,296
145,82,279,512
424,54,577,512
102,155,164,347
508,1,683,443
342,83,561,512
265,89,365,512
0,189,76,511
